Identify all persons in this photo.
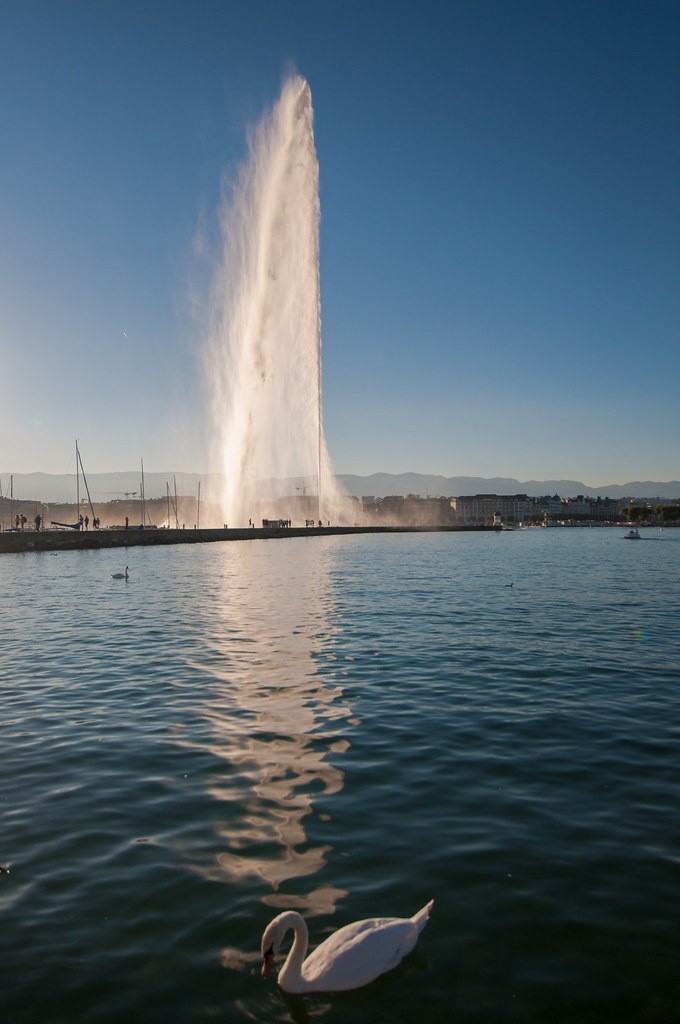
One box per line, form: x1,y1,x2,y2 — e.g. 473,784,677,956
14,513,27,528
79,515,100,532
34,513,42,532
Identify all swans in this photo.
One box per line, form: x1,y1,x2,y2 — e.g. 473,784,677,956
259,899,435,993
111,565,130,579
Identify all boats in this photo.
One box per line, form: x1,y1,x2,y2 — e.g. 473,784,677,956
4,524,158,532
624,529,641,538
